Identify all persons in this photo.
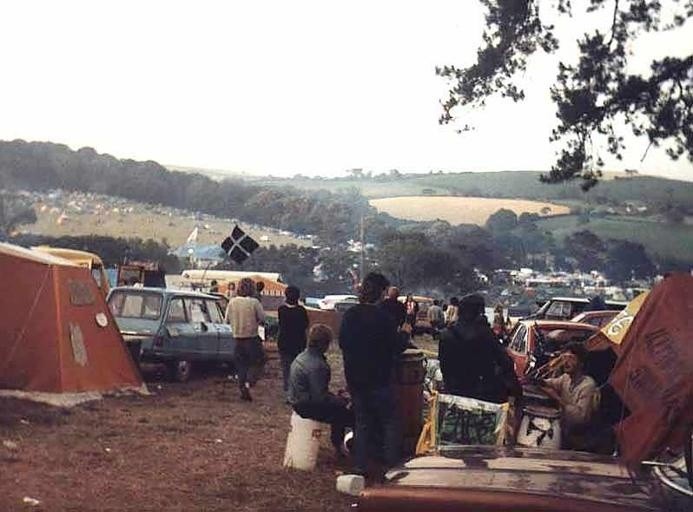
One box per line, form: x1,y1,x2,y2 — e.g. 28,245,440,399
108,276,150,317
286,323,354,469
224,277,266,401
339,273,414,469
277,285,309,391
182,274,265,303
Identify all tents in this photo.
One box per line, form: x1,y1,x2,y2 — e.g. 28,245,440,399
1,240,152,411
527,270,693,461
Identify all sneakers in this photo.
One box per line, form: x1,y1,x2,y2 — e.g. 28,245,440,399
240,384,252,401
384,465,410,482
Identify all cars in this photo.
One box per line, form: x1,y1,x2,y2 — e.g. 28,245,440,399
105,284,233,382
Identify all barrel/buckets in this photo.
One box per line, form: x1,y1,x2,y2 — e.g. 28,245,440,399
283,412,321,471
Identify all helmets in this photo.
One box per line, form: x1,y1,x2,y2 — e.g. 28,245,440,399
309,323,333,342
